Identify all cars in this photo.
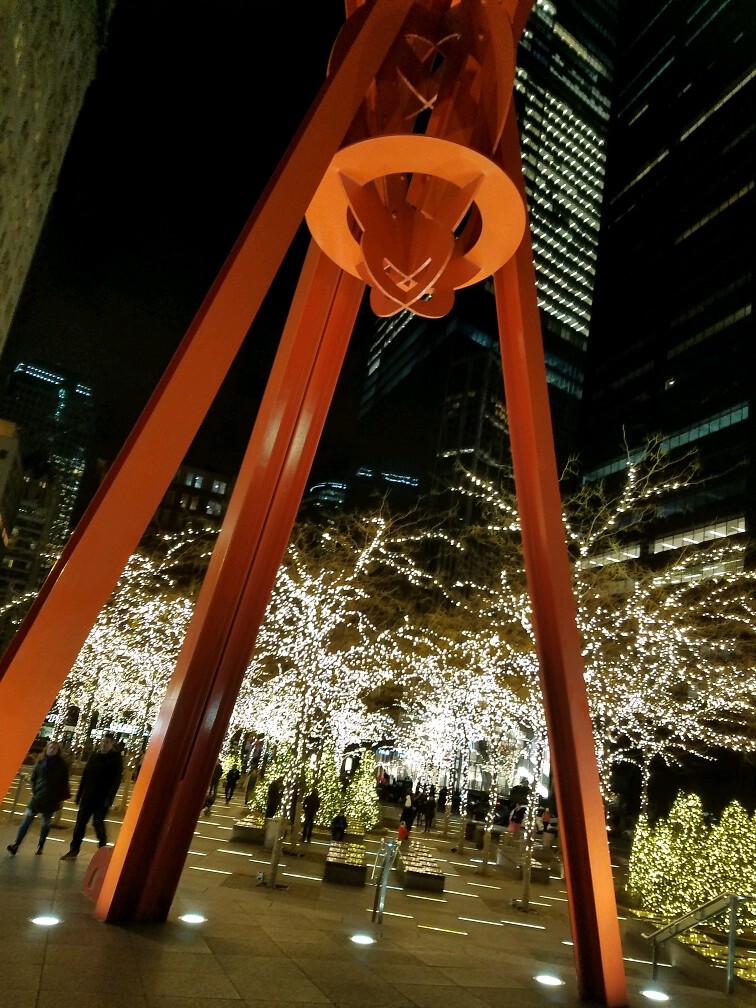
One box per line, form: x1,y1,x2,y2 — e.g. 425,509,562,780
509,806,557,833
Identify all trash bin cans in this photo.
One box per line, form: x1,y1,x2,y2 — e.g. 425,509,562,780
475,825,501,851
466,821,488,842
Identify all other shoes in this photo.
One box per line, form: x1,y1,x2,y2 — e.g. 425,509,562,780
61,851,77,860
35,845,43,855
7,844,18,855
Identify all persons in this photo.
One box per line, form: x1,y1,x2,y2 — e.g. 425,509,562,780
331,809,348,842
9,741,70,856
62,733,122,859
242,769,259,805
397,776,460,840
300,789,321,843
209,759,224,795
542,808,550,831
511,803,525,833
225,765,241,805
266,777,285,818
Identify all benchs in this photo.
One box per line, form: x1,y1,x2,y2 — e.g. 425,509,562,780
323,841,367,888
232,812,265,844
393,840,446,892
490,840,548,882
